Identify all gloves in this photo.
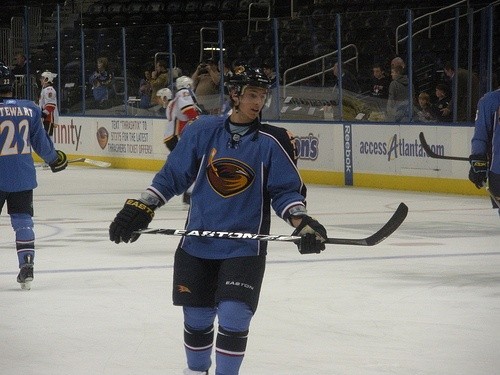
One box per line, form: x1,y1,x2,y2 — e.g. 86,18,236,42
109,198,155,244
468,154,488,190
290,215,327,254
50,150,69,173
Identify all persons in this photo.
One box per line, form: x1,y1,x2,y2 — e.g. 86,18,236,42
11,52,484,124
164,75,204,205
468,88,500,218
39,71,56,170
0,63,69,290
110,65,327,375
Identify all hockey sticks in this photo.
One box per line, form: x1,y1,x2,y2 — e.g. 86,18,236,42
418,131,470,161
139,202,408,244
34,154,112,170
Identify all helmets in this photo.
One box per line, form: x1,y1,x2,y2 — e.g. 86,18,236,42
176,76,194,91
223,59,272,96
41,71,54,84
0,64,17,94
156,87,174,101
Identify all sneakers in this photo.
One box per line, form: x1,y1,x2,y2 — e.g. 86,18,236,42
17,265,33,290
